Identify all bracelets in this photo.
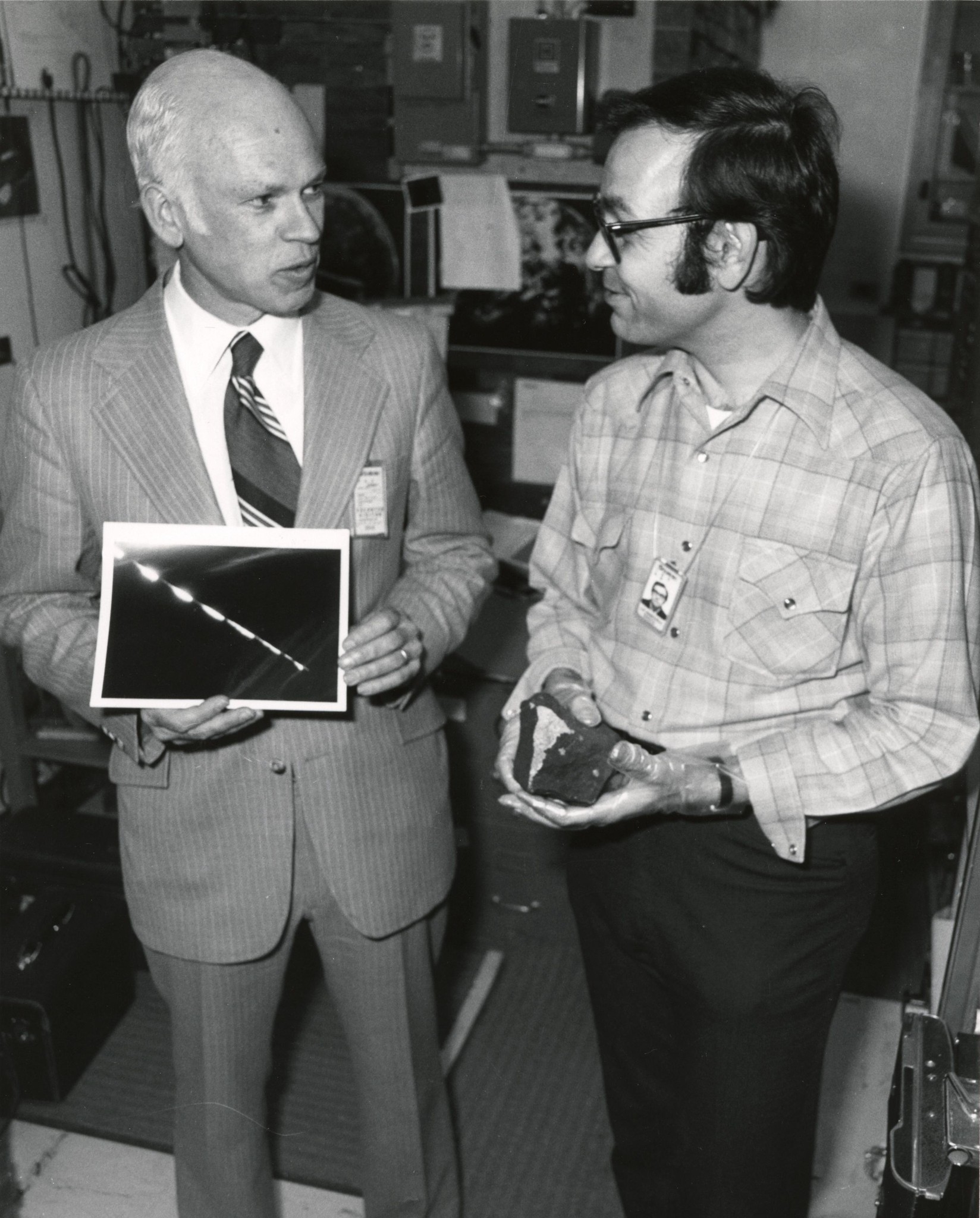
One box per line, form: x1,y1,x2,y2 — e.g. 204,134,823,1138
708,753,735,818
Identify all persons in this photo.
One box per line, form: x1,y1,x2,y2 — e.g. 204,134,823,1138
492,76,980,1218
0,45,500,1217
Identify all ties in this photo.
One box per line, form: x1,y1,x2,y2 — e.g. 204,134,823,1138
223,330,304,529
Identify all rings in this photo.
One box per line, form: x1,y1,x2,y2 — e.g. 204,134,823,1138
399,649,411,664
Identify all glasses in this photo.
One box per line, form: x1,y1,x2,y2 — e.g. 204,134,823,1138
590,192,770,266
